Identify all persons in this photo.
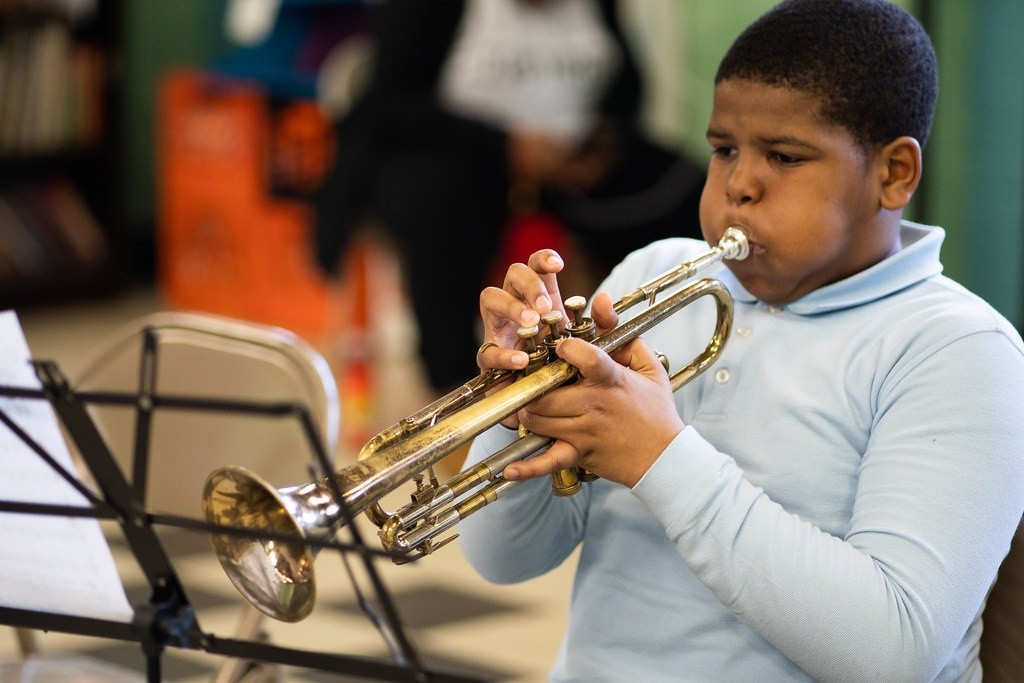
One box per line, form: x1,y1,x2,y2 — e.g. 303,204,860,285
219,0,709,431
453,1,1024,683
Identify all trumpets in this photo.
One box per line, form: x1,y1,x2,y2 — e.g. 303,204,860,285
202,223,751,624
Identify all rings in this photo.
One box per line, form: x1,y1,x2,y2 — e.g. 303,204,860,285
478,342,499,369
